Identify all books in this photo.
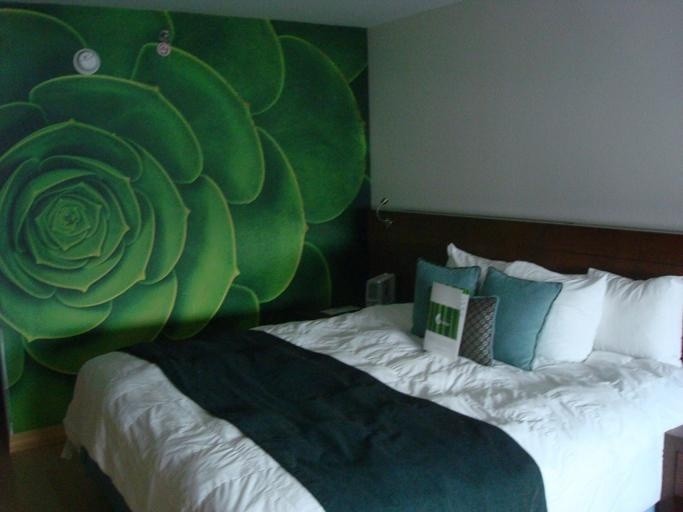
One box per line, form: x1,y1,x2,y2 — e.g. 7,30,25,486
421,280,471,362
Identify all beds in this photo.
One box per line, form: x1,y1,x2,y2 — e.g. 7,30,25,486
67,302,682,509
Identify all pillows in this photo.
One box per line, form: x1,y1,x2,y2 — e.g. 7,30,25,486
412,238,683,374
